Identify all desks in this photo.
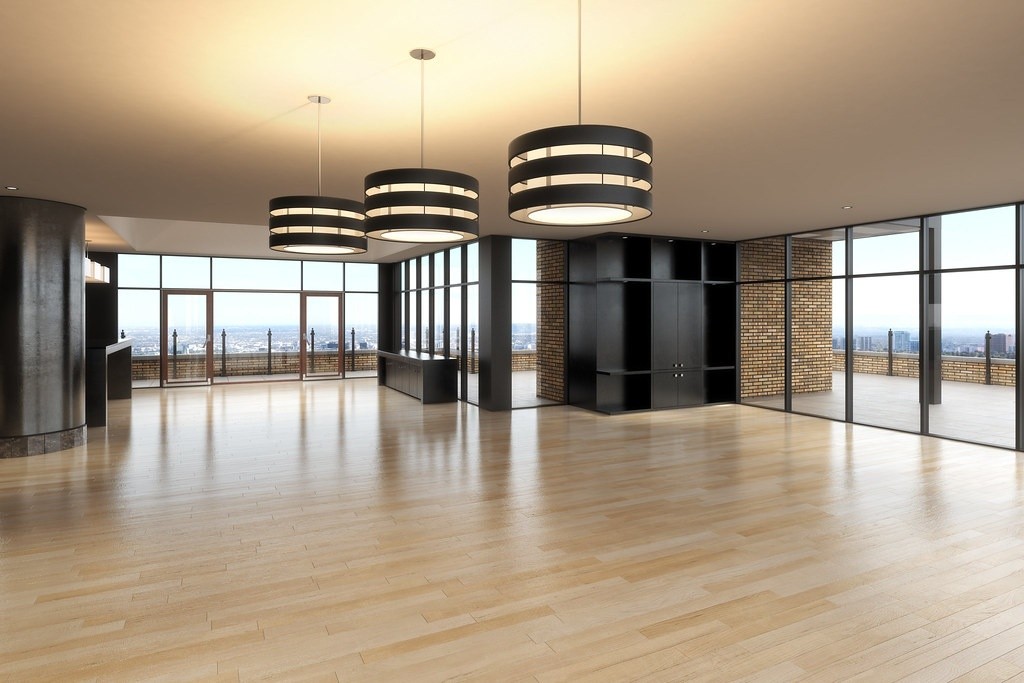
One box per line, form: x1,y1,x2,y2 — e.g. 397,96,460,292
86,336,134,428
377,349,459,405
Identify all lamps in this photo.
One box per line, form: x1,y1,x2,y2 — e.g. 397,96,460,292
84,240,110,285
508,0,654,227
269,96,368,255
364,49,479,243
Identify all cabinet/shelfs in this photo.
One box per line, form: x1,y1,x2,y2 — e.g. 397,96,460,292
566,231,739,416
